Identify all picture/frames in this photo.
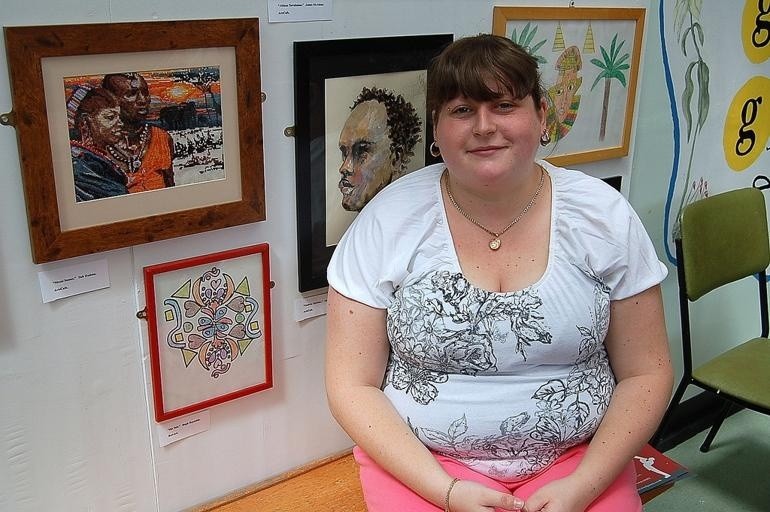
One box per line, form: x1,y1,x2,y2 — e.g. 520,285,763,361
2,17,267,266
492,4,648,168
143,246,274,423
294,33,454,294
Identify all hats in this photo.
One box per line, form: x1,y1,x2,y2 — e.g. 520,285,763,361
66,83,96,129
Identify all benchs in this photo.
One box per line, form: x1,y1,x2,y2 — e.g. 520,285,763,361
183,437,688,509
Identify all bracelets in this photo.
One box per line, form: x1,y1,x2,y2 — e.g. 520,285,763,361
444,478,460,512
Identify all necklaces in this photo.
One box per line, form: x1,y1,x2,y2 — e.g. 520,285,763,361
444,168,548,251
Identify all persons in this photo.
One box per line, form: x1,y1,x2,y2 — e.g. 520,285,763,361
338,85,417,215
65,72,175,203
325,34,676,512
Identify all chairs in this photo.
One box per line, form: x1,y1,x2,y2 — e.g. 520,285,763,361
654,184,770,451
